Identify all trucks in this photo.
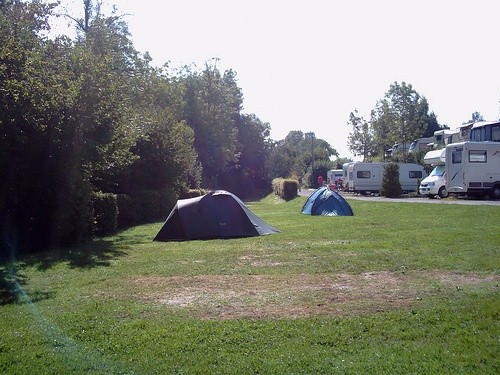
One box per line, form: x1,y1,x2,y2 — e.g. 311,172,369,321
327,161,430,197
384,118,500,158
444,141,500,201
418,147,449,199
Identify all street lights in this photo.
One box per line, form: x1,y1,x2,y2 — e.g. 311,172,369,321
310,132,315,169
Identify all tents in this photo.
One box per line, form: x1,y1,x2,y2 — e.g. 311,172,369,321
301,186,354,216
153,190,281,242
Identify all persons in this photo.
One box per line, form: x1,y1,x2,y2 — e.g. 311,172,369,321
318,174,324,187
345,182,349,194
298,176,302,192
337,177,343,189
